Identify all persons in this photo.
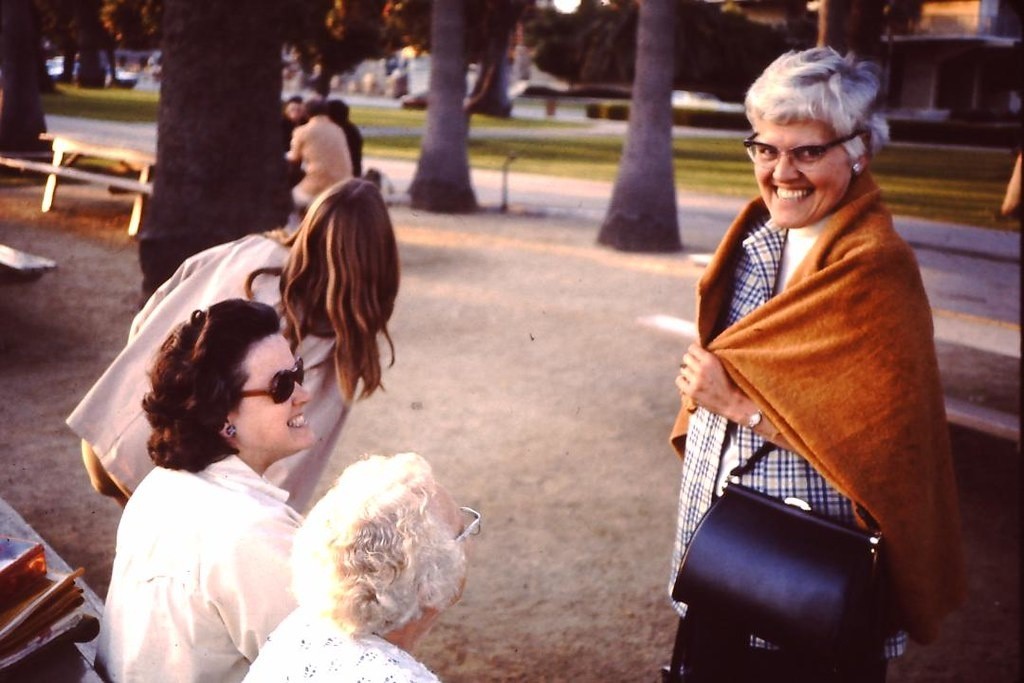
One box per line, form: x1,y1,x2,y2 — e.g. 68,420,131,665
1001,151,1024,217
283,95,363,207
97,298,314,683
241,451,481,683
66,177,401,516
665,45,965,683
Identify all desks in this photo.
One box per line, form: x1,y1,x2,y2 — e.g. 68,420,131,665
39,134,157,237
691,254,1020,359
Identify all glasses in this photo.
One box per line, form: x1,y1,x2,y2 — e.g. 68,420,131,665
241,358,306,403
455,507,481,543
744,133,863,164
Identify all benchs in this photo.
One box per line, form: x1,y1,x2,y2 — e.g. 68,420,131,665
0,157,153,196
651,212,1021,443
0,244,58,282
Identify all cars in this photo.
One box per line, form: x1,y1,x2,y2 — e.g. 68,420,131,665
45,51,161,91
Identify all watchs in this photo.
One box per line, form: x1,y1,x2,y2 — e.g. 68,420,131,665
745,411,762,431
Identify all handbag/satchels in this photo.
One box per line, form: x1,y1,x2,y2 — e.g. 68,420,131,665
670,482,892,662
